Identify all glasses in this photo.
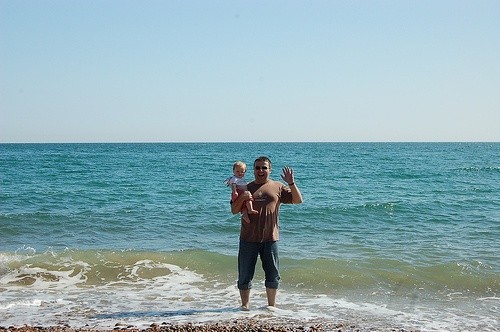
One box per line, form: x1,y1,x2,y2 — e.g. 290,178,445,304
255,166,269,170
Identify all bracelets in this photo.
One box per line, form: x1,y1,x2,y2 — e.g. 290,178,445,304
288,183,295,185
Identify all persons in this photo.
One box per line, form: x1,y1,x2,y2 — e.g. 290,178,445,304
230,162,259,225
229,156,303,307
